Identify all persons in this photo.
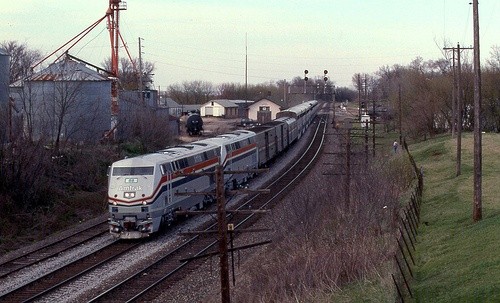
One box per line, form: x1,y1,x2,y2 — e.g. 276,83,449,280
393,140,398,152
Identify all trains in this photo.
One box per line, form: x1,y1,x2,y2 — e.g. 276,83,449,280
107,99,320,239
